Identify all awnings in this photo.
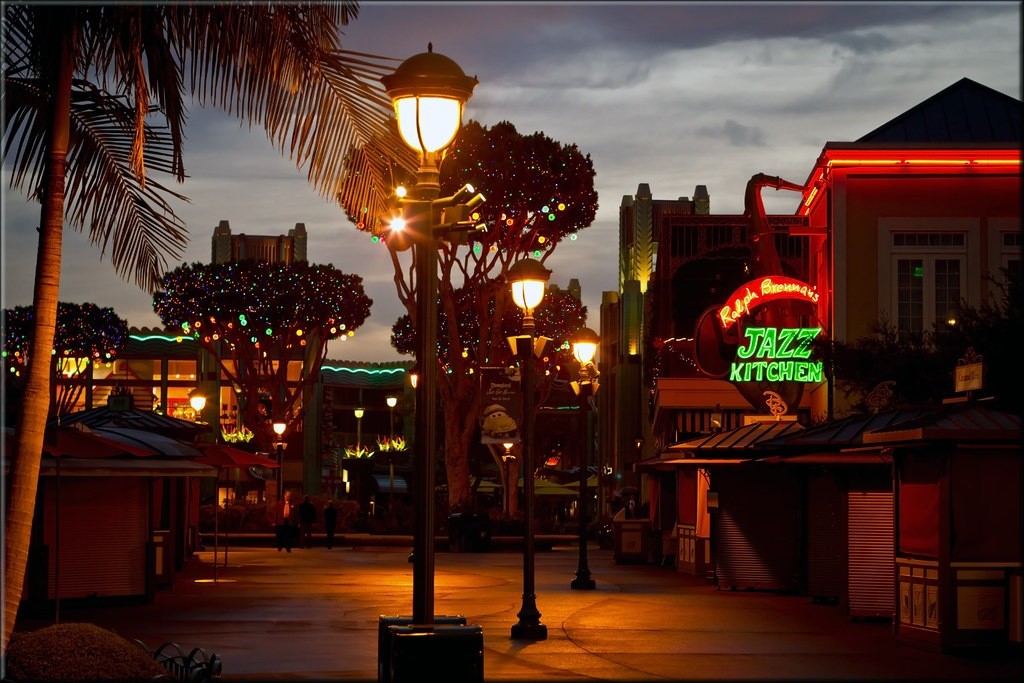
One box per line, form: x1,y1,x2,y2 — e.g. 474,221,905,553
193,442,282,468
372,475,407,493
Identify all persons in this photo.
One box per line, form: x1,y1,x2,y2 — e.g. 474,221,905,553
324,501,337,549
447,501,492,552
299,495,316,548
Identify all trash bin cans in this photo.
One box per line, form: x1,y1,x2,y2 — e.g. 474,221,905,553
447,512,491,552
612,519,649,564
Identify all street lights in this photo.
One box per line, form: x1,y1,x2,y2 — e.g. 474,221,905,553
502,251,553,642
566,324,602,593
383,394,396,512
272,421,288,548
378,34,484,631
354,404,367,457
186,389,206,423
502,434,515,525
